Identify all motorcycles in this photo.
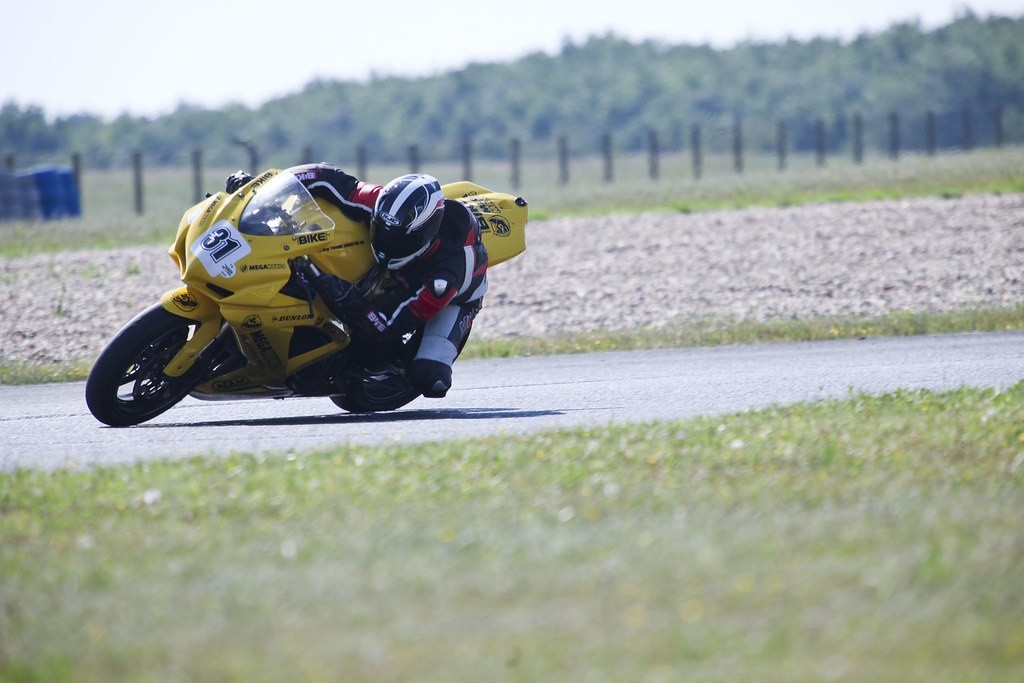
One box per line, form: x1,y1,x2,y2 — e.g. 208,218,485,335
85,166,528,428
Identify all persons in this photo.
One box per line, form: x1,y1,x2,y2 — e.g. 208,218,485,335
226,162,489,398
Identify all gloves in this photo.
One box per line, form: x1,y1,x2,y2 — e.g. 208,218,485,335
289,255,374,321
225,171,258,193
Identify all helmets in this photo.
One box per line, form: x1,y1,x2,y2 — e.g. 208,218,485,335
371,173,445,270
